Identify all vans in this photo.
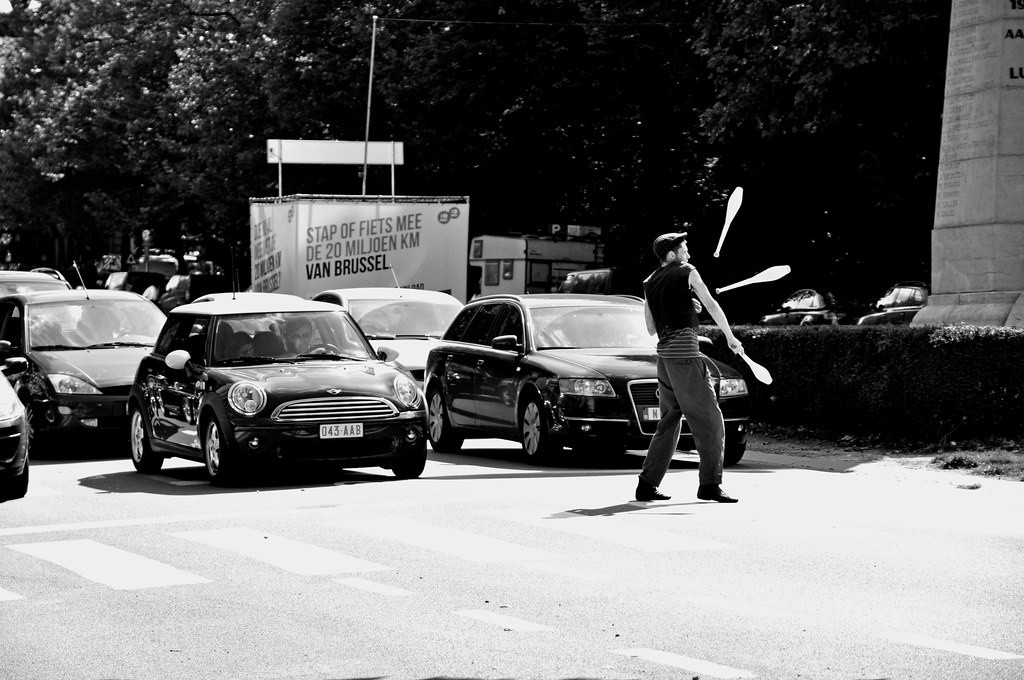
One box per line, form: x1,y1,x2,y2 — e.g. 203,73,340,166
104,271,166,294
163,274,233,305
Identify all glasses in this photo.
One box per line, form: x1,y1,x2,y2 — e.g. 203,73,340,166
293,332,310,340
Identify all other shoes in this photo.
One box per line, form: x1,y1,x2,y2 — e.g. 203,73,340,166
697,484,738,503
635,487,671,501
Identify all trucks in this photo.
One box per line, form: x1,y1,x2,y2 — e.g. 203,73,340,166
468,233,606,302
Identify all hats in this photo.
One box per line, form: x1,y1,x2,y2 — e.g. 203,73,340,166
652,232,687,257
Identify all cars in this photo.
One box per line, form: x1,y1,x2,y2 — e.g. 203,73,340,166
312,287,466,391
142,285,166,304
0,288,168,456
1,371,29,503
758,278,930,328
127,294,431,490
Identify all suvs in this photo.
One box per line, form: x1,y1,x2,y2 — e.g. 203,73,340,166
1,266,73,298
557,269,623,294
422,292,751,469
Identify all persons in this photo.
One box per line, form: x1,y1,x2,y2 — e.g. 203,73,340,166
223,332,254,360
635,232,742,503
275,317,312,359
